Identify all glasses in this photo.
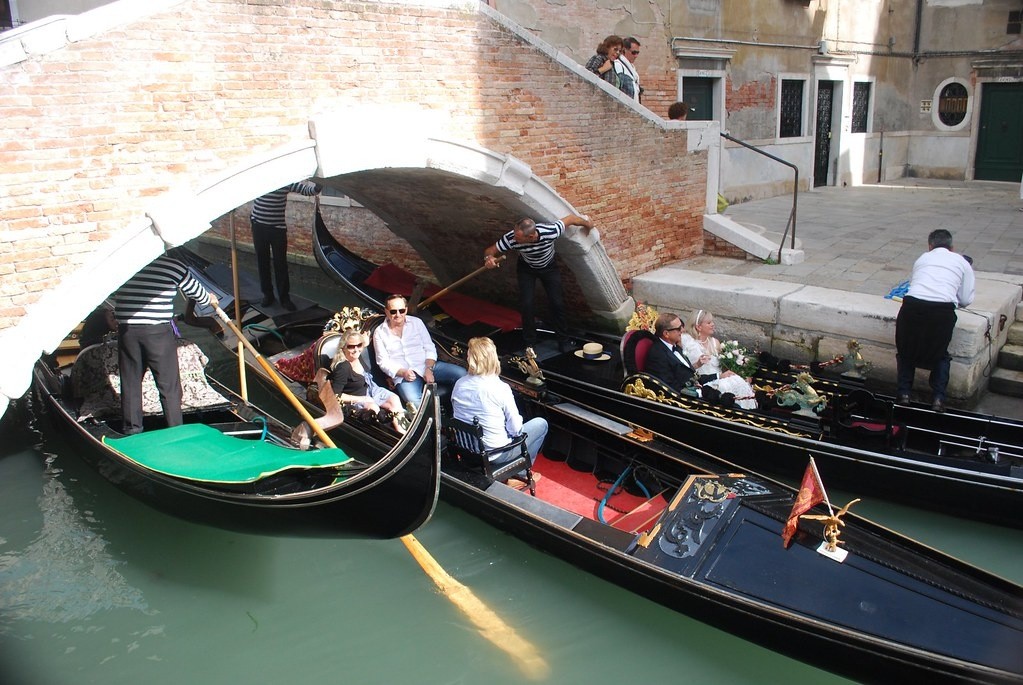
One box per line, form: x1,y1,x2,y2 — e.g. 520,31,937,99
666,325,683,332
346,343,363,349
628,48,641,55
613,48,622,52
385,307,406,315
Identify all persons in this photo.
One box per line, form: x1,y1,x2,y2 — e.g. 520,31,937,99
248,181,324,312
113,253,220,436
484,214,597,337
373,294,467,409
585,35,642,105
643,310,759,411
451,337,548,490
329,330,407,436
668,101,689,121
895,229,977,413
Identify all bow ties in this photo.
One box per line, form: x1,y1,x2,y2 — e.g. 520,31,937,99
672,345,680,352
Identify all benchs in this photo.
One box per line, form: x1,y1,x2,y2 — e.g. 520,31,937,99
56,322,85,350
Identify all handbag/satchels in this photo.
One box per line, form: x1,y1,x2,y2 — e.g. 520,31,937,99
614,61,633,98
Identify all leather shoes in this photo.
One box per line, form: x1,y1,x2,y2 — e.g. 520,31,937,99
894,392,911,405
932,399,947,412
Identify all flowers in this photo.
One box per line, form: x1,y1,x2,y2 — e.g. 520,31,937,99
716,340,759,379
829,388,907,452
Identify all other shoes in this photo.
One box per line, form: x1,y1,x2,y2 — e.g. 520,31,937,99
263,294,276,308
507,472,542,490
280,299,297,311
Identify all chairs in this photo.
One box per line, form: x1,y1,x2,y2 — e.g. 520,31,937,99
619,327,654,374
316,332,347,375
440,405,537,496
362,314,451,403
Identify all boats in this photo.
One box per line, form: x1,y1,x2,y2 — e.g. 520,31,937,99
307,194,1023,528
166,242,1023,685
23,294,443,539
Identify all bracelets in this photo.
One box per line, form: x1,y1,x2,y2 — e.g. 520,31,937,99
484,255,488,260
